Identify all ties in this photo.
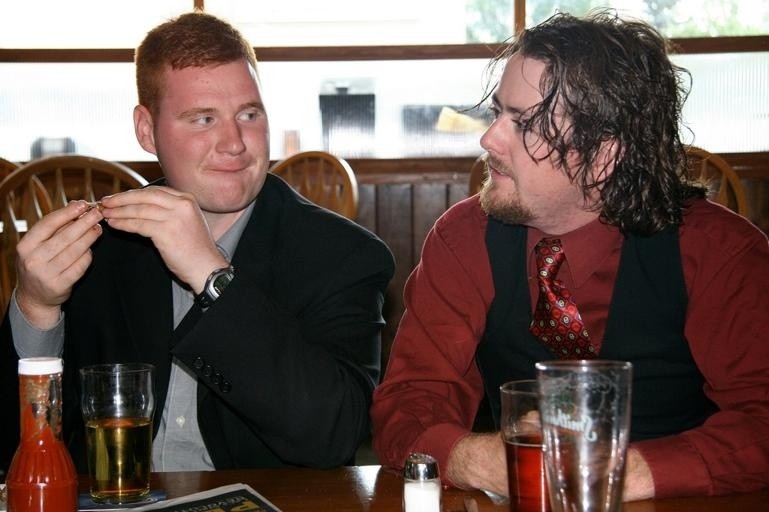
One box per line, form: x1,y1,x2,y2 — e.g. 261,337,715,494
529,236,597,360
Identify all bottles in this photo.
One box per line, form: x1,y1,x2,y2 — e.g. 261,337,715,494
8,355,76,512
400,454,443,512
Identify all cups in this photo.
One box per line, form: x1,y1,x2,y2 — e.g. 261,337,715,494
78,361,159,505
534,360,634,509
501,380,574,511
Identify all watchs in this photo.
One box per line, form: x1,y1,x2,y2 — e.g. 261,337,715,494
197,265,239,309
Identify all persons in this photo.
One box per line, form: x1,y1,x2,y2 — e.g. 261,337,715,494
0,11,395,471
371,16,768,505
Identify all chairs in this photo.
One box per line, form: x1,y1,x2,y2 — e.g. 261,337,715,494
467,142,753,221
